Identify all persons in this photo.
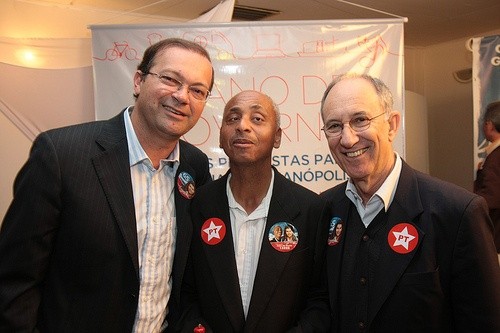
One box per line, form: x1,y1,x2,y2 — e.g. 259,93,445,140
330,221,342,243
321,73,500,333
473,102,500,253
271,226,298,244
190,92,320,333
180,181,195,198
0,38,214,332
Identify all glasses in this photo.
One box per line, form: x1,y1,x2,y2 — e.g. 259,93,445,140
138,69,211,102
320,108,388,137
